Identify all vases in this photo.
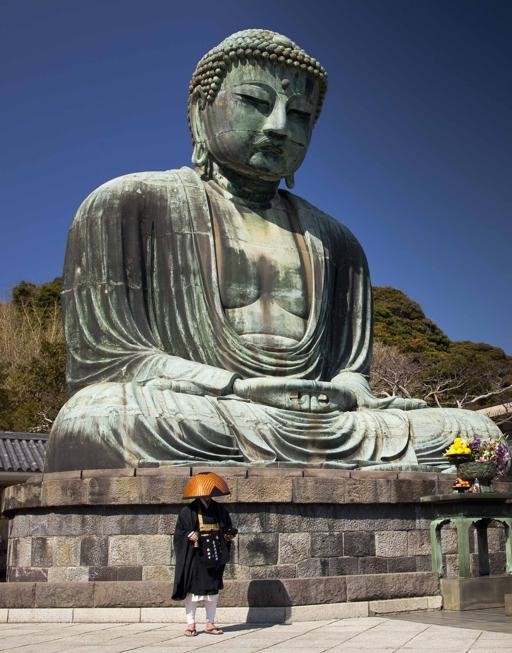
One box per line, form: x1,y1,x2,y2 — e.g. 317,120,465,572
458,462,497,493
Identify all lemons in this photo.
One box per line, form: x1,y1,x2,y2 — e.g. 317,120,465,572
447,438,471,455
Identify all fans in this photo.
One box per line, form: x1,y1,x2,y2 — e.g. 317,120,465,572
467,429,512,480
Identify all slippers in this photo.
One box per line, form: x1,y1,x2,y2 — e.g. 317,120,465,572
204,628,224,634
184,629,197,637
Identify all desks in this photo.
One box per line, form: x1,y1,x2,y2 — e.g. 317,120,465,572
419,492,512,577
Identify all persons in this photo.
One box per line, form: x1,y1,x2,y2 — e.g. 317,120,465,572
169,494,240,637
45,25,512,478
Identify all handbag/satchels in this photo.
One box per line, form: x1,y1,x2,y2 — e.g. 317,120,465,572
203,535,230,568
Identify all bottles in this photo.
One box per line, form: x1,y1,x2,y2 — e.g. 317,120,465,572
473,477,480,493
